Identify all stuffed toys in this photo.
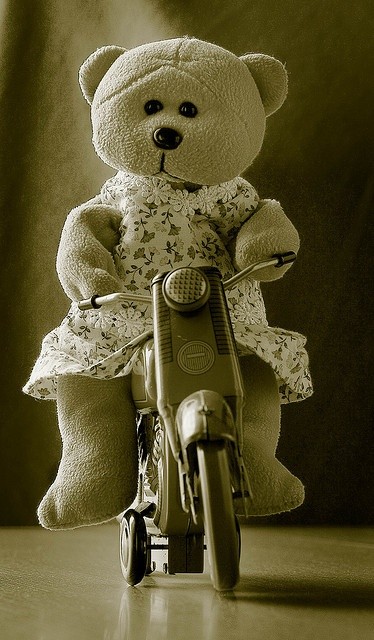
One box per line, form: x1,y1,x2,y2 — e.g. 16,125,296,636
22,36,316,532
